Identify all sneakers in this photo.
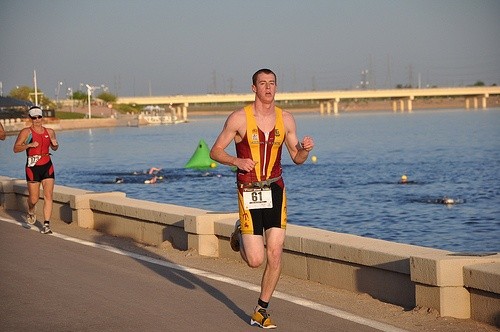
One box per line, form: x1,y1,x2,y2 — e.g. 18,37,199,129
250,308,277,328
230,219,242,252
27,205,36,224
41,225,52,234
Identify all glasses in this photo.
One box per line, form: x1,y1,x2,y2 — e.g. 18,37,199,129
29,115,42,119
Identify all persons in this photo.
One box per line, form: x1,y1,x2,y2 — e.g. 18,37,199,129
14,106,59,234
209,69,314,328
0,122,6,140
134,166,164,185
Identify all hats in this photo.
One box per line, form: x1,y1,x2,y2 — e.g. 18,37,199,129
29,109,42,116
